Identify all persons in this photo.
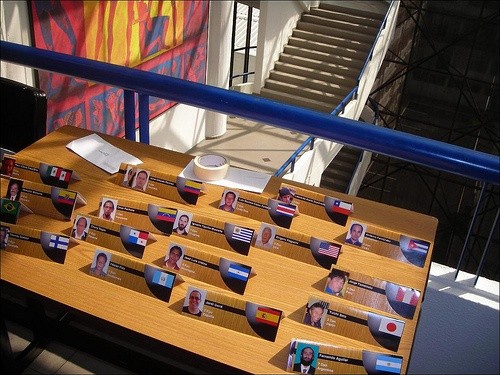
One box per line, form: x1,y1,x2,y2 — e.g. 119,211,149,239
325,272,346,297
256,226,272,248
293,347,315,374
72,217,88,241
7,183,20,201
90,253,107,278
122,169,134,186
172,215,189,236
133,170,148,191
163,245,182,270
306,302,324,329
182,290,202,317
278,188,292,203
100,200,115,221
219,191,236,212
345,224,364,247
2,158,14,176
1,229,6,249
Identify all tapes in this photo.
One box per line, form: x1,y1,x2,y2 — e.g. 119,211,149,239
192,152,230,180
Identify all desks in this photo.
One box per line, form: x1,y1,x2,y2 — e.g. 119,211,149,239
0,125,439,374
